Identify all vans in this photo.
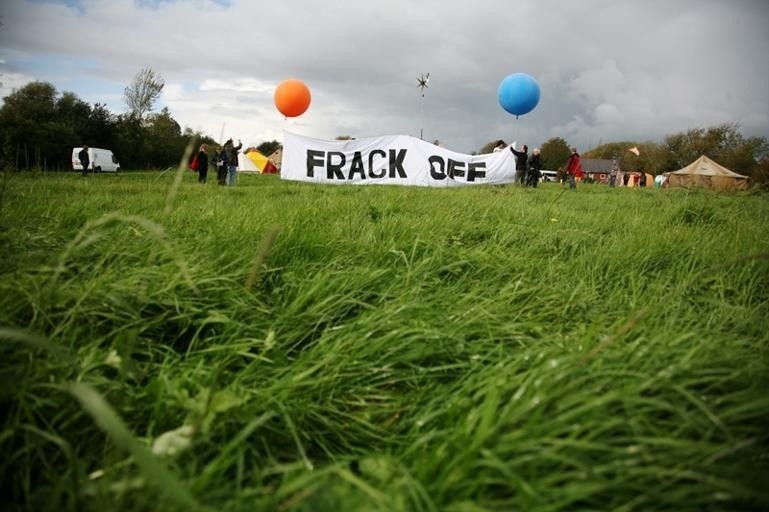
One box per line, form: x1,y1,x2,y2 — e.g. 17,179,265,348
539,170,557,182
71,147,121,173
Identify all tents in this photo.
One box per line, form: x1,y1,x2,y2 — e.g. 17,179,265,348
234,150,260,174
246,147,278,175
659,153,752,194
267,145,284,175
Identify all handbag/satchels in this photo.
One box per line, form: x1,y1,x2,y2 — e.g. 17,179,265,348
191,155,201,170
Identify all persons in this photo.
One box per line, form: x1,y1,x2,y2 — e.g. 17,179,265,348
196,143,209,184
78,145,89,176
509,143,528,187
565,146,585,193
223,138,243,186
492,139,507,190
632,173,640,188
211,144,227,186
524,147,544,188
639,171,646,189
623,172,630,186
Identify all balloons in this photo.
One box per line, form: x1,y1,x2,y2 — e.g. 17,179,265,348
273,77,311,121
496,70,542,121
